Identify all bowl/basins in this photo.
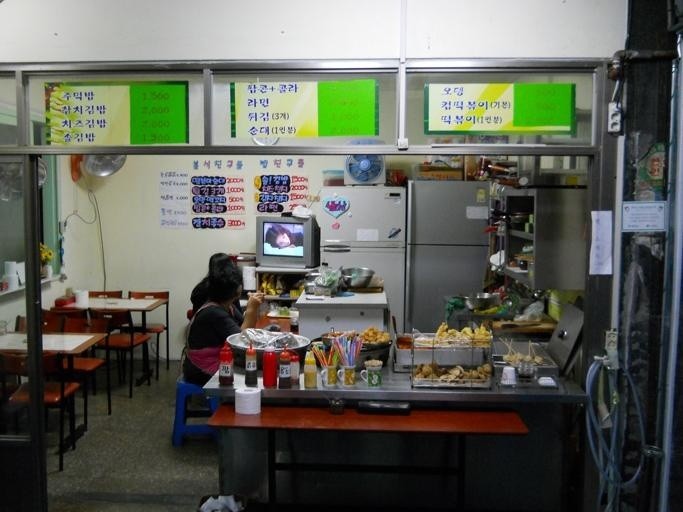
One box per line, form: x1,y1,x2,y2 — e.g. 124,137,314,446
226,328,311,368
457,292,499,310
338,267,376,289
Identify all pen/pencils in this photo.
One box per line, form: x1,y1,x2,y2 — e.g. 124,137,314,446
333,336,361,367
312,345,340,368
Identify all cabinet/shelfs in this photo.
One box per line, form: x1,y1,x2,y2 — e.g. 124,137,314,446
490,188,588,291
255,266,309,327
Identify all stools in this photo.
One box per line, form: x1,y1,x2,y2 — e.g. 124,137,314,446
172,375,221,449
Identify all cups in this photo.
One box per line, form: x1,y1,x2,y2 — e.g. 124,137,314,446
501,363,517,386
318,366,338,387
359,367,383,388
337,364,356,388
72,288,89,310
2,260,26,291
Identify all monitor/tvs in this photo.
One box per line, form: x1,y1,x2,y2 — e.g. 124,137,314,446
256,217,320,269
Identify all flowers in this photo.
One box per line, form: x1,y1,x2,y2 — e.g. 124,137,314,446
40,243,53,268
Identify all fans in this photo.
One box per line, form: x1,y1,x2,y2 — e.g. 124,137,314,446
70,154,126,182
344,138,385,186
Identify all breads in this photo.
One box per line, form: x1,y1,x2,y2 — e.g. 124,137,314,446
413,361,496,383
432,319,493,348
362,326,391,345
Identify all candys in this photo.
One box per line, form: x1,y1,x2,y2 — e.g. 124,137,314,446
501,337,544,367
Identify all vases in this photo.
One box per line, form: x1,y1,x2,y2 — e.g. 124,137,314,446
40,262,47,278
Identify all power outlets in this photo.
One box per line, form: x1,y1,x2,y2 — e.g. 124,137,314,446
59,221,67,232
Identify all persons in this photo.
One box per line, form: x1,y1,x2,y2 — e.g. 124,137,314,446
182,266,264,392
191,253,244,326
265,225,303,250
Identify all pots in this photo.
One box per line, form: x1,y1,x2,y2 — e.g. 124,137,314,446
356,340,392,368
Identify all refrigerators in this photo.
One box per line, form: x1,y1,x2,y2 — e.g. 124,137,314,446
318,184,406,334
403,179,494,333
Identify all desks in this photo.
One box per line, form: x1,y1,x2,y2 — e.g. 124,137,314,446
207,405,529,512
255,311,291,333
296,288,387,339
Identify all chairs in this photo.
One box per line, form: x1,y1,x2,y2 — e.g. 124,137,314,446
0,291,169,471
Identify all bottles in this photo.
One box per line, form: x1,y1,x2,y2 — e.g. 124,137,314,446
530,290,546,314
216,340,316,389
